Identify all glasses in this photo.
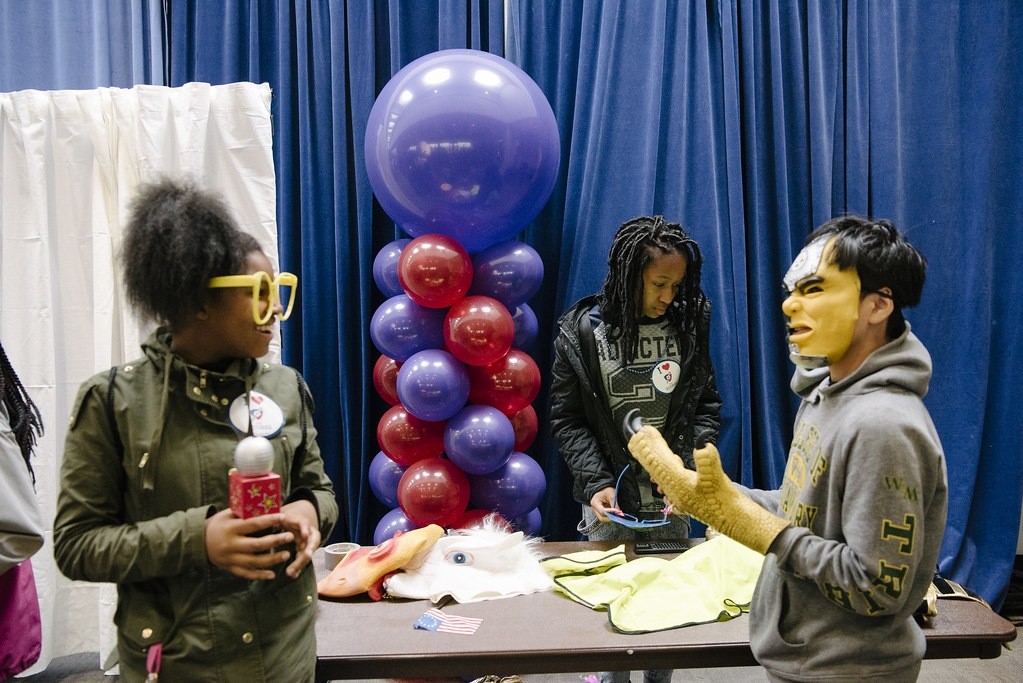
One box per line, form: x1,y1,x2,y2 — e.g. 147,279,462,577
208,271,298,325
604,464,671,530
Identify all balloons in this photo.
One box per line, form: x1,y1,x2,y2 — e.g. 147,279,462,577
369,233,546,544
365,48,561,253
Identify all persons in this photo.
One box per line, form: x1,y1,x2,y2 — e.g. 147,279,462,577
629,217,948,683
550,216,723,683
0,345,44,579
54,180,337,683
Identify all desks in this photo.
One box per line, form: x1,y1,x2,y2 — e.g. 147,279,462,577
312,542,1018,683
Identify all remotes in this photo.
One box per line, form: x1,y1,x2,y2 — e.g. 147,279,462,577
634,541,690,554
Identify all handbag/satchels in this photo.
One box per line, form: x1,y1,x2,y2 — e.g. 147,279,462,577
0,556,42,681
